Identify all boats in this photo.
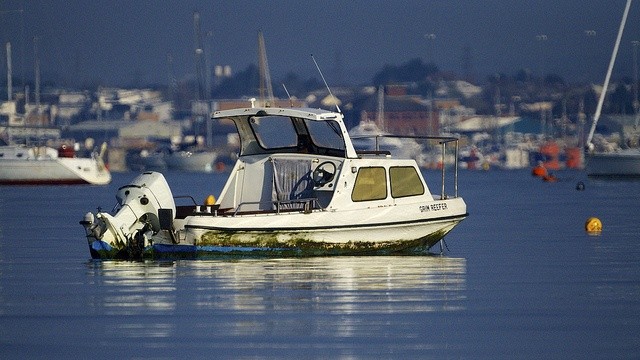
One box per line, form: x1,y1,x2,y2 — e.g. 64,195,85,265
77,54,468,259
581,0,640,177
5,41,587,176
0,37,114,189
